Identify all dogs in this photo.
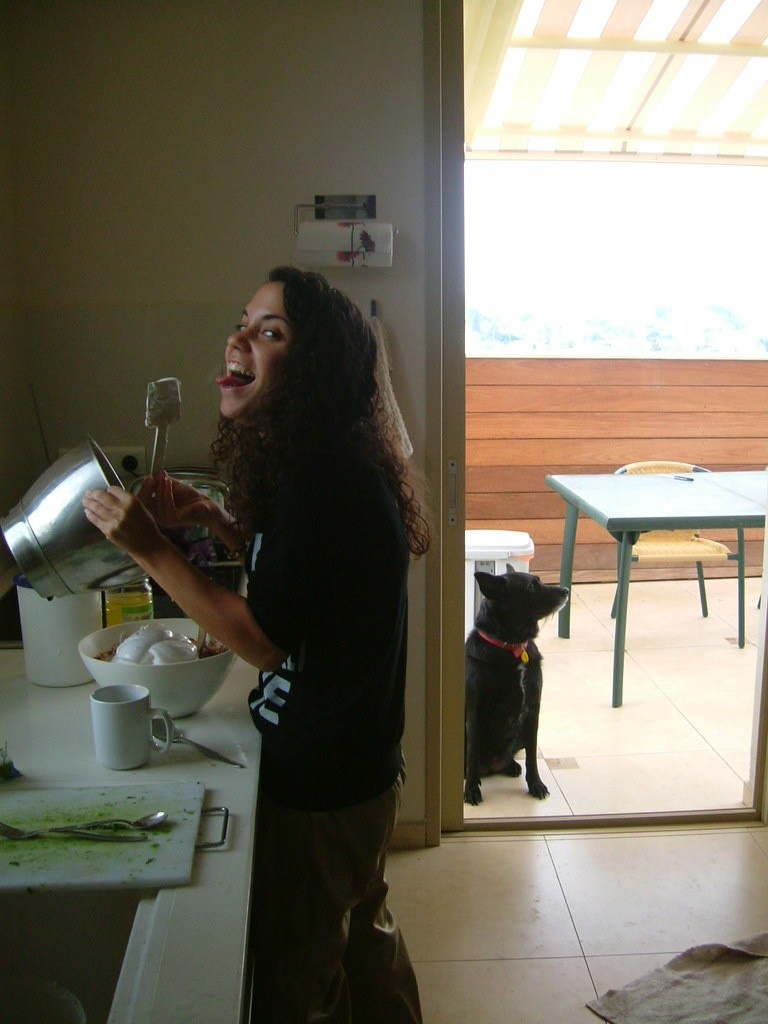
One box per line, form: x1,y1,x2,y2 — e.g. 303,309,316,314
465,562,570,808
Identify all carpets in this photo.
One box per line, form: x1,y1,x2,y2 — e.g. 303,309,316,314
584,932,768,1023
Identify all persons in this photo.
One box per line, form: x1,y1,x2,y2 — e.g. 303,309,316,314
80,265,434,1023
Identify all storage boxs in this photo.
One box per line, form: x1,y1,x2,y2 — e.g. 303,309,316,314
464,529,536,642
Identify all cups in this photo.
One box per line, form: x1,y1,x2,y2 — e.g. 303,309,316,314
90,684,172,770
17,572,104,687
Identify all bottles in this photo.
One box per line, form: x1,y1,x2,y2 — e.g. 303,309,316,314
105,577,153,627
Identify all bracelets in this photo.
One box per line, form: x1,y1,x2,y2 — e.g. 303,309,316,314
223,548,242,560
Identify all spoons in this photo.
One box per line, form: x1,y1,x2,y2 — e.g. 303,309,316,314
0,822,147,842
49,811,168,832
151,719,244,769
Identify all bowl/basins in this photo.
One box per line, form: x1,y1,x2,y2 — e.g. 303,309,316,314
0,437,148,599
79,618,239,718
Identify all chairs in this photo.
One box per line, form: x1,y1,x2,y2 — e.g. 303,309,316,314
610,460,745,649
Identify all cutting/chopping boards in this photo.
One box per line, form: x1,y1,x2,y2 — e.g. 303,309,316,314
0,782,229,897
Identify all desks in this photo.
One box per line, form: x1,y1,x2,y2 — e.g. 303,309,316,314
545,471,768,709
